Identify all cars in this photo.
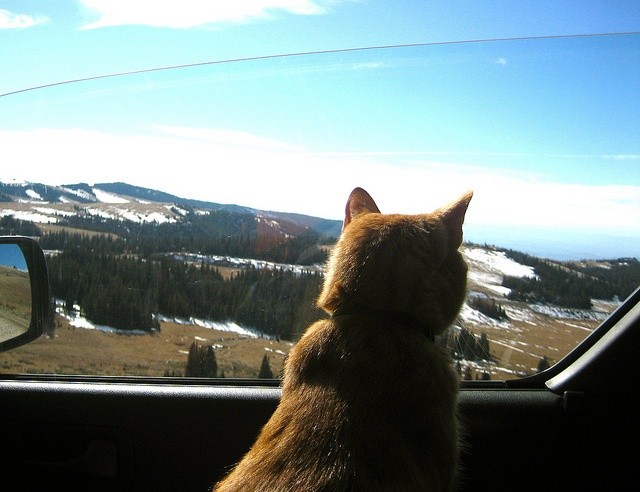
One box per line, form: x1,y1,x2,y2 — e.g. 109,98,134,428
0,237,637,487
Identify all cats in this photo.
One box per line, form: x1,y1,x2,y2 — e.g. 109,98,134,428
212,186,474,492
